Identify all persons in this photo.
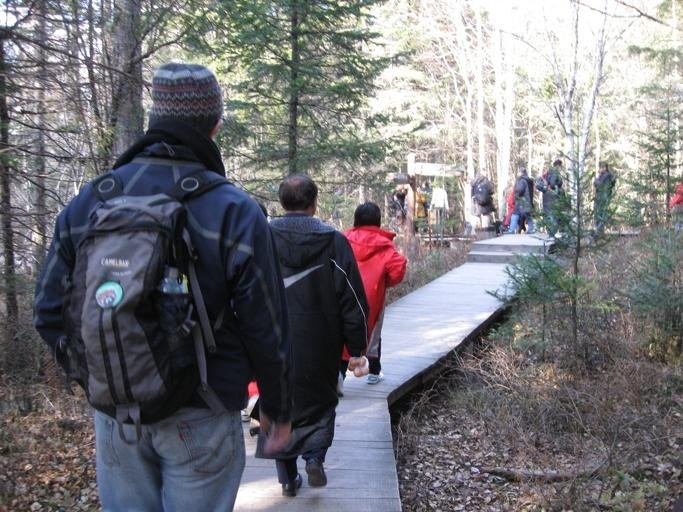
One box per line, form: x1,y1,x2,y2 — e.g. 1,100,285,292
469,158,565,236
389,179,451,234
34,62,291,512
590,161,617,236
260,171,372,497
668,178,683,233
240,199,270,436
343,199,406,386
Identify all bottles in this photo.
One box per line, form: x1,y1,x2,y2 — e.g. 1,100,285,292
158,267,185,330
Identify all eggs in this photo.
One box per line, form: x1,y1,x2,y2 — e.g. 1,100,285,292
346,356,372,377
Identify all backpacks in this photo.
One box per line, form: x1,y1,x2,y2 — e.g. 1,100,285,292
535,172,549,192
59,170,227,426
475,184,491,205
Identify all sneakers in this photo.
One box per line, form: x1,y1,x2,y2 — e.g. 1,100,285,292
305,457,327,486
366,372,383,385
502,230,514,234
337,372,345,397
282,472,302,495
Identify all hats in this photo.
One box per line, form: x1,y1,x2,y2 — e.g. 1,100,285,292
148,63,222,133
